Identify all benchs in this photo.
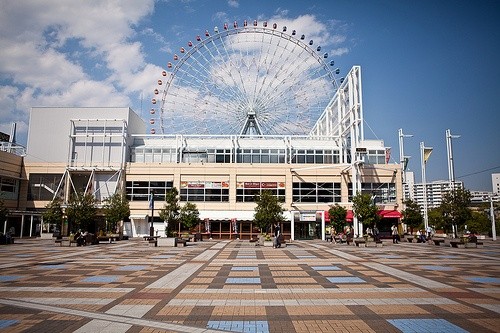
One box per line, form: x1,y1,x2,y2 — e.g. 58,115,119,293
55,241,61,246
432,238,445,245
71,240,77,246
477,242,483,248
286,243,299,247
404,236,420,242
450,242,465,248
177,240,185,247
327,235,380,247
149,241,157,247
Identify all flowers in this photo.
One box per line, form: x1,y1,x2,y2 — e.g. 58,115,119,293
266,235,272,241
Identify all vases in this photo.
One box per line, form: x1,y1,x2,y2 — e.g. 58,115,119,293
264,241,273,247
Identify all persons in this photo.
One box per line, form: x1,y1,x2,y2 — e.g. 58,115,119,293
391,223,399,244
329,226,336,244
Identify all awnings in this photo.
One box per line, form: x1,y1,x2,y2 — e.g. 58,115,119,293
324,210,402,222
172,210,292,222
